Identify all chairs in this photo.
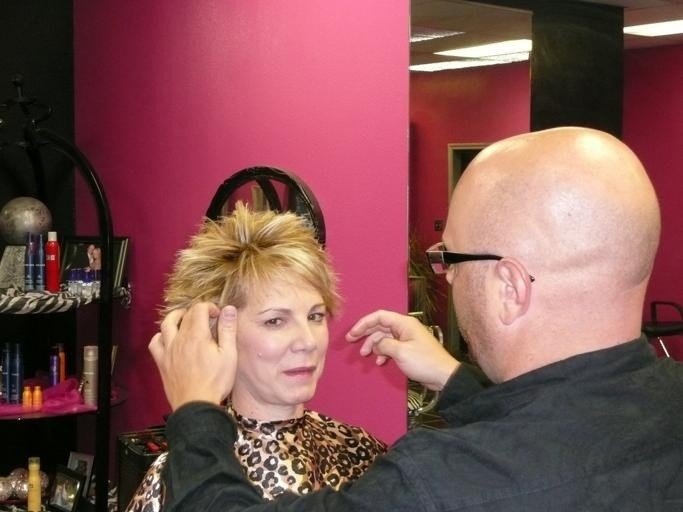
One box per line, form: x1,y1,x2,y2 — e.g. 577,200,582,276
643,301,682,359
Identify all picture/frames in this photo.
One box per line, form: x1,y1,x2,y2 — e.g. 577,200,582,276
62,237,129,293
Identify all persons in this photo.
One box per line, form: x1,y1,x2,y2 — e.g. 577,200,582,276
148,124,683,511
53,479,70,505
126,200,388,511
78,243,101,272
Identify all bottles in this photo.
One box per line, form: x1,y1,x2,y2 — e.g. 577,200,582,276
44,232,60,293
49,342,65,386
21,387,40,404
81,343,96,404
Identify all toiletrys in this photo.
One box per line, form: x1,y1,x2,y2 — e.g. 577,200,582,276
82,345,99,407
33,386,42,406
22,387,33,408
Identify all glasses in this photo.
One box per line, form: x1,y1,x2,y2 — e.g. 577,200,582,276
426,242,535,282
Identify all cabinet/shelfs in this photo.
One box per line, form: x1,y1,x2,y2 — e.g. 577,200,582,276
0,129,128,511
